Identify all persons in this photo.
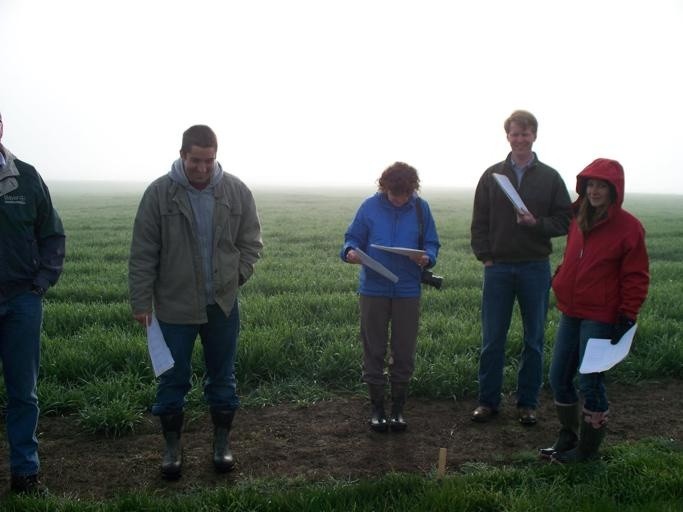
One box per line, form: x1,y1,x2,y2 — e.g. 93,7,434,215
128,125,262,479
539,158,650,467
340,162,440,430
467,111,572,426
0,113,66,495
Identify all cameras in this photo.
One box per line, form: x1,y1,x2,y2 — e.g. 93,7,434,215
421,270,444,290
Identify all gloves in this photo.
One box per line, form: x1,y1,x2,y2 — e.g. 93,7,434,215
610,312,636,345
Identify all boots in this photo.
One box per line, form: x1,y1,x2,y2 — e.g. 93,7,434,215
368,382,389,433
208,405,236,473
548,402,610,467
158,410,184,483
538,396,582,459
389,382,409,432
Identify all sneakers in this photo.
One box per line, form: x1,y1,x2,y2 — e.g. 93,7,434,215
517,405,539,425
470,404,499,423
11,472,49,497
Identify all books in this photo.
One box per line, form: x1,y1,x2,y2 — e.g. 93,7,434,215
492,172,532,216
